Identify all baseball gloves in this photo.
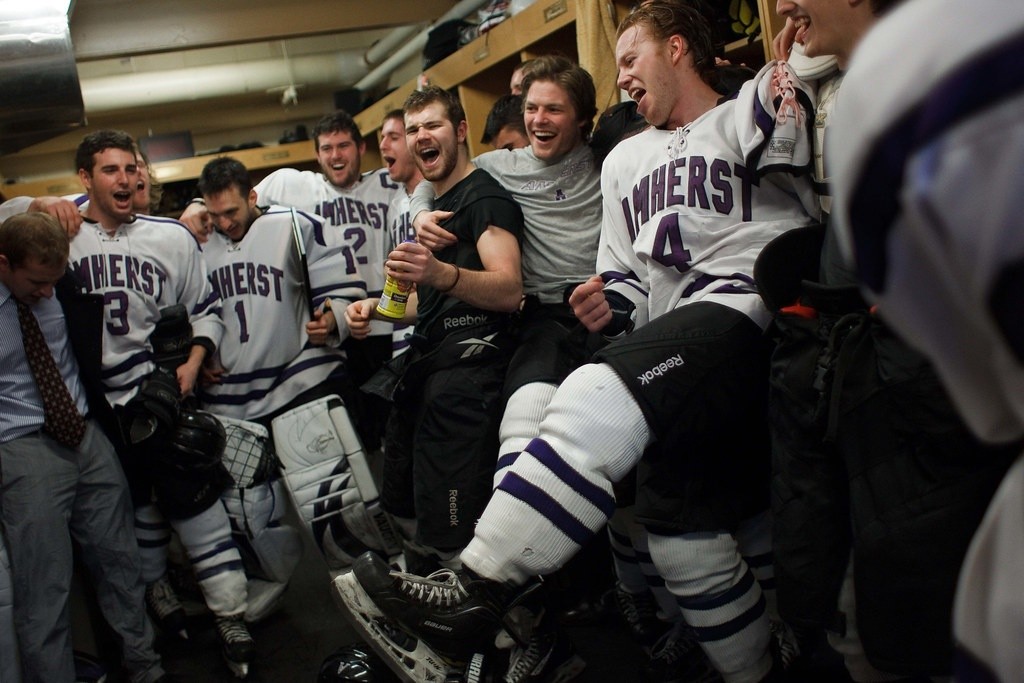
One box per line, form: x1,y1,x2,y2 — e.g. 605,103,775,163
126,364,184,446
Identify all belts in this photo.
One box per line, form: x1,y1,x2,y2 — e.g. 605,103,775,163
29,411,92,437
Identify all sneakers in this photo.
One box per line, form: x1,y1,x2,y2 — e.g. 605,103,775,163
637,619,706,683
329,552,523,683
777,624,817,675
214,616,256,678
496,599,587,683
246,573,290,619
146,574,190,639
601,582,668,654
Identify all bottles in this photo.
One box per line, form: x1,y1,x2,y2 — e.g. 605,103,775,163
375,239,417,322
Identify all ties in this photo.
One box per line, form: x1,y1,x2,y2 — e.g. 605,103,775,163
9,295,86,449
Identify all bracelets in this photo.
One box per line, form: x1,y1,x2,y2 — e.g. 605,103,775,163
441,264,459,292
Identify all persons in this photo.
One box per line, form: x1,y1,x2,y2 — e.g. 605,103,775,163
0,0,1024,682
